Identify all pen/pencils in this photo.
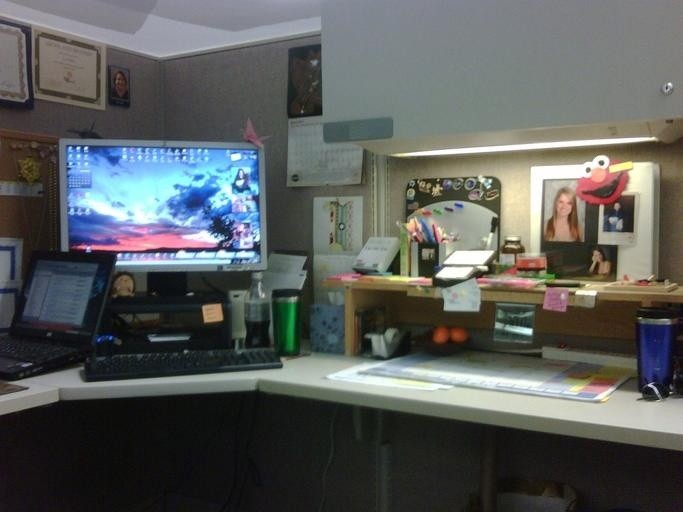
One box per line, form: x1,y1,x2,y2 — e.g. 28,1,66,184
545,281,584,287
401,216,454,243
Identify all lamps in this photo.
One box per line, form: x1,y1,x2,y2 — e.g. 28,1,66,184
353,117,683,158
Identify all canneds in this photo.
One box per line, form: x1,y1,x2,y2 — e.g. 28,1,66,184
499,235,523,264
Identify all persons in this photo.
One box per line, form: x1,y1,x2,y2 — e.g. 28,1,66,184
542,186,580,243
110,69,129,99
232,168,250,193
604,200,625,232
589,247,613,276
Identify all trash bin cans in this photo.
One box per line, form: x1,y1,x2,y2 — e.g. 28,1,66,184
496,477,578,512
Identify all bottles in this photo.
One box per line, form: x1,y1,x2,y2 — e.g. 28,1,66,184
245,269,270,346
500,235,525,268
273,289,301,356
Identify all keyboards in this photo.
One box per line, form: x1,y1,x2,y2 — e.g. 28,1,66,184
85,349,283,383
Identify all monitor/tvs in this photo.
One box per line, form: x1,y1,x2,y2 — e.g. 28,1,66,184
58,137,268,312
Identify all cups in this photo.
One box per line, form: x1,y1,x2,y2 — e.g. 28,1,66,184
636,306,679,396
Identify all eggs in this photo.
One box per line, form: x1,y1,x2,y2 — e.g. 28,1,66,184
432,325,449,345
450,327,469,342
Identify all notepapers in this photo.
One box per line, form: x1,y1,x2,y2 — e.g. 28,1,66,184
327,273,434,285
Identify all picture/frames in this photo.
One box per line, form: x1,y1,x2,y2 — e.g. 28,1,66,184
108,65,130,108
0,17,34,111
530,162,660,282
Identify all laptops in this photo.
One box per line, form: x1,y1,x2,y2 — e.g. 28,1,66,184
0,250,117,381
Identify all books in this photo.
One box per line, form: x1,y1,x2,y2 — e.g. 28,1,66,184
349,237,399,273
